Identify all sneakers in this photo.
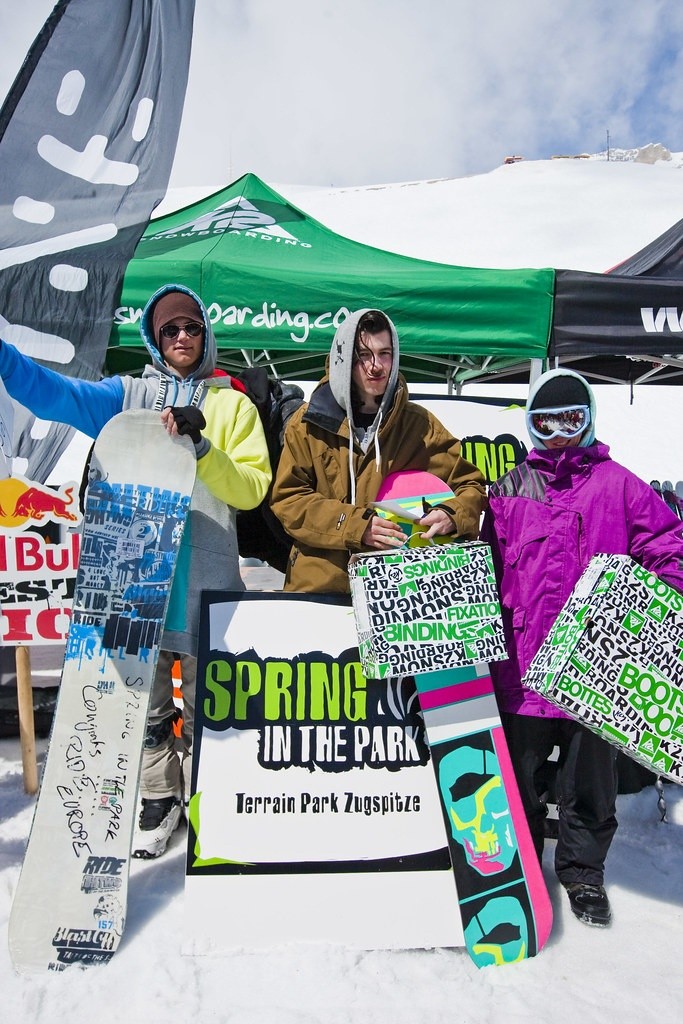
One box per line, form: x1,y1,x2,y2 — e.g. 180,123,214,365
131,796,182,860
562,879,612,927
183,800,190,823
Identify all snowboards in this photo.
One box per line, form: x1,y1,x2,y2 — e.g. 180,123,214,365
8,406,198,985
371,470,556,968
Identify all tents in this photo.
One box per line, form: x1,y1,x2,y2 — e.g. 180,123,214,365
0,174,683,395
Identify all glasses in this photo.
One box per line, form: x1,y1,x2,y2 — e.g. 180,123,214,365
527,404,591,440
160,322,205,339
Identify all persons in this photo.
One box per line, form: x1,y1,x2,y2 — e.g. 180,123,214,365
480,368,683,927
270,308,487,594
0,283,273,860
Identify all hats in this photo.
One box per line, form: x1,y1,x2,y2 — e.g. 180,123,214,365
529,375,591,411
150,291,206,351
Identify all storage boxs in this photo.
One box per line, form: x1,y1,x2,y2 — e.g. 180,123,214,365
347,537,510,680
519,551,683,787
